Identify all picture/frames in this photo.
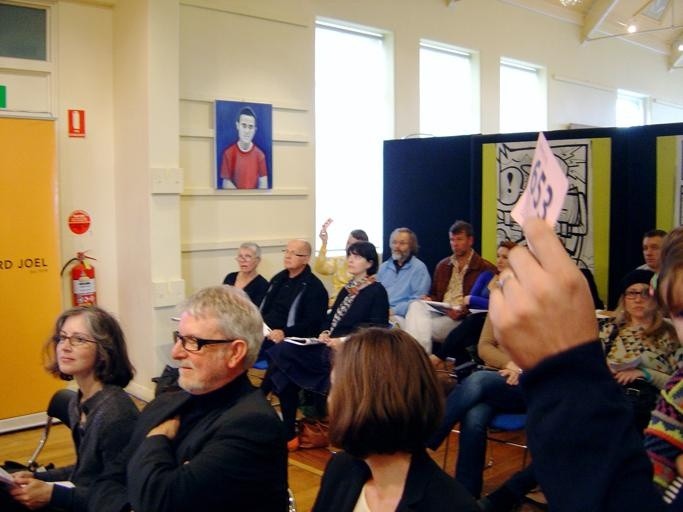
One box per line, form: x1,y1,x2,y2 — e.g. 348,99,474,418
214,99,273,191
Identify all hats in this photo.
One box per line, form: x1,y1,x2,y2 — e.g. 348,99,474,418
622,269,655,293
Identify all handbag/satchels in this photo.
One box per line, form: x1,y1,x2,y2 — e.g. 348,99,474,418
298,422,329,449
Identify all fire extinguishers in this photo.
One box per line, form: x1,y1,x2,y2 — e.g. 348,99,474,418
60,250,98,308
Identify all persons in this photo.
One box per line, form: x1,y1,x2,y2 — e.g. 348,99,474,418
310,324,488,512
87,285,287,512
0,305,141,511
220,106,269,190
222,221,500,450
489,218,683,512
423,230,679,512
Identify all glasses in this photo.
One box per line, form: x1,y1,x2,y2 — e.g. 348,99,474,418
626,291,650,299
282,249,306,256
173,331,233,351
55,331,97,346
236,256,253,262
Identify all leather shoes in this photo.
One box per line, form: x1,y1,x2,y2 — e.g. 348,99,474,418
286,436,299,450
